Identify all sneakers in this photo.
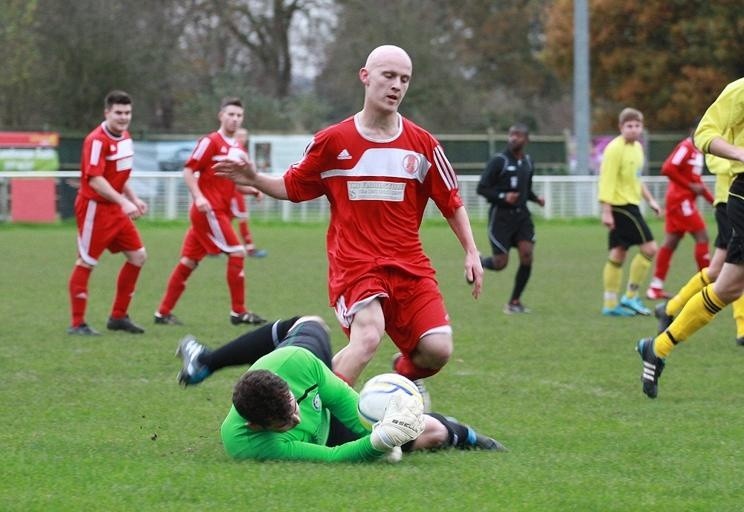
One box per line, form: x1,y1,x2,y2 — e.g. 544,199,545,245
230,312,266,325
621,296,651,317
153,312,182,326
603,305,636,317
68,324,102,338
107,315,144,334
638,337,666,397
655,304,674,336
453,417,506,448
176,333,211,385
646,286,673,298
391,352,432,413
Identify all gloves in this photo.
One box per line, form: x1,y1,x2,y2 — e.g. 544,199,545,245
375,394,427,448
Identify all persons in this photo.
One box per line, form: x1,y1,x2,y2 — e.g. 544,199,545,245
154,95,268,326
212,43,484,416
67,90,146,334
208,128,267,257
598,106,663,316
465,122,545,316
635,76,744,398
177,315,510,465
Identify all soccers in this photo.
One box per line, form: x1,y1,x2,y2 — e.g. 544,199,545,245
355,373,425,432
